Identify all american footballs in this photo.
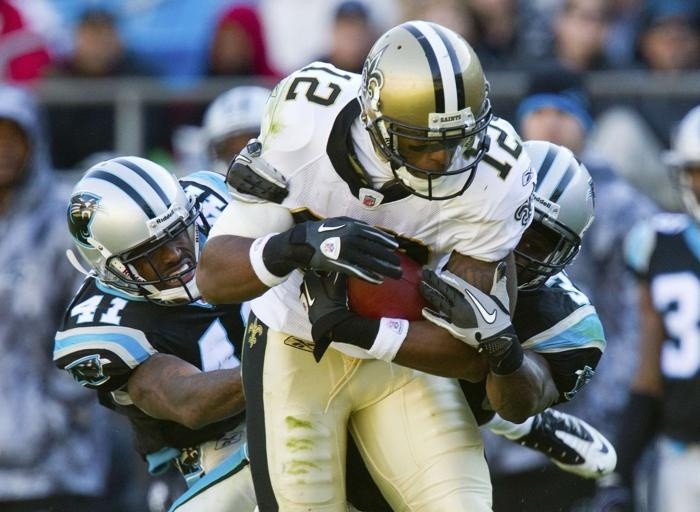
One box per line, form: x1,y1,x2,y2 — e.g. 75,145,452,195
348,252,425,320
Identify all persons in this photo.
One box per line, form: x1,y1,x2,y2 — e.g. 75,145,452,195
310,3,696,509
196,20,538,508
52,155,262,511
172,137,607,508
2,3,286,510
203,85,619,477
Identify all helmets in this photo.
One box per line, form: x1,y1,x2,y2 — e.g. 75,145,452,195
0,87,42,145
517,138,597,299
65,155,202,310
201,86,274,173
661,106,700,228
356,21,494,201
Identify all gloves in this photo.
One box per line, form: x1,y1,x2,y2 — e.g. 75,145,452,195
289,217,403,286
417,269,517,357
300,269,349,362
622,392,664,461
223,138,289,205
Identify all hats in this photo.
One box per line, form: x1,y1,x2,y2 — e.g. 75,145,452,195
516,65,595,130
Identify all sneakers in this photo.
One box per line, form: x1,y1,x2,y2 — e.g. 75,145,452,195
504,407,618,478
